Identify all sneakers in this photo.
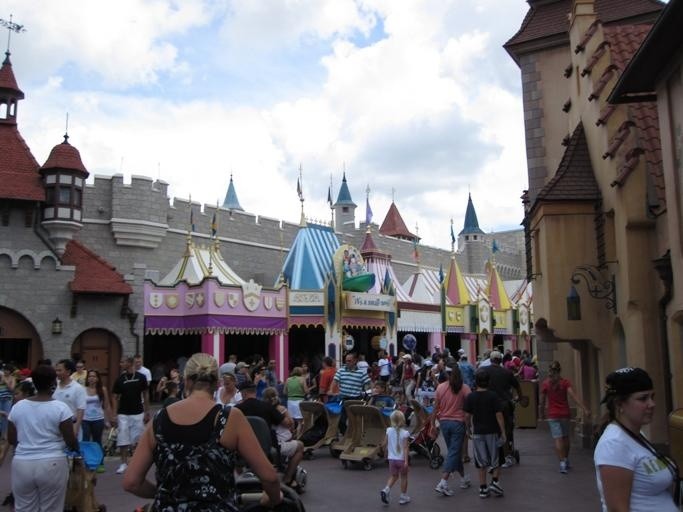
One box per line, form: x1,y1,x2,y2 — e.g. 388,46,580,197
116,463,128,474
380,455,570,504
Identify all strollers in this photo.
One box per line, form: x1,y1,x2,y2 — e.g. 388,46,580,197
66,454,108,511
299,397,444,471
475,393,530,469
236,476,306,511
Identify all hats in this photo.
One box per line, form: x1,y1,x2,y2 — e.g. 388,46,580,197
599,368,651,406
489,350,504,359
236,381,255,393
236,362,249,369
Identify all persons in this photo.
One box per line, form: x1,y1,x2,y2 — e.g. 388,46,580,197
122,352,285,511
462,371,508,500
537,360,593,473
5,362,82,512
1,355,192,474
427,362,476,497
592,367,683,512
213,348,537,481
378,410,412,506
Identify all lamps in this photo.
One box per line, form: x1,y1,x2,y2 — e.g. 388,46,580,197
51,315,62,336
568,260,619,316
